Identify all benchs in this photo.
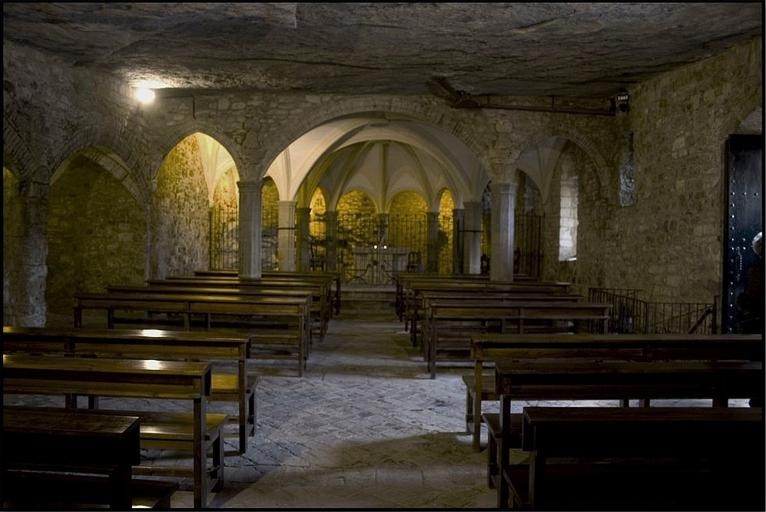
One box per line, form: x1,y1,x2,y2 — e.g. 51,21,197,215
462,333,764,453
2,353,230,510
2,405,187,511
3,325,262,454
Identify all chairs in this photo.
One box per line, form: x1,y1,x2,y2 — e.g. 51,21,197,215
72,271,343,379
502,404,764,511
481,358,764,511
394,268,615,380
